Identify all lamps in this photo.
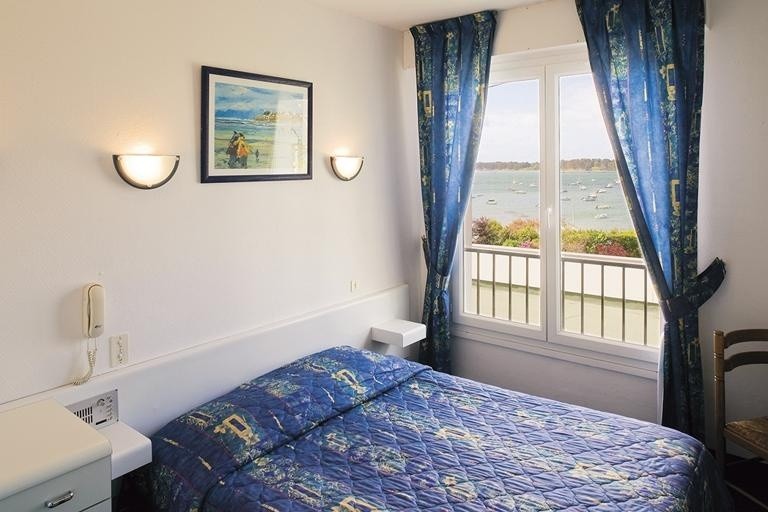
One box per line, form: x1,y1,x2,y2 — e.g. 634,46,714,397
112,154,180,189
330,155,364,181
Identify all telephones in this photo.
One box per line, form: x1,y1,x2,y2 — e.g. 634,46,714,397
83,282,108,337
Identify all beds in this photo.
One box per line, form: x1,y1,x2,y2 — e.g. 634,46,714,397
115,345,726,512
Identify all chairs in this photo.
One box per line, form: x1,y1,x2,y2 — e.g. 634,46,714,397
711,327,767,511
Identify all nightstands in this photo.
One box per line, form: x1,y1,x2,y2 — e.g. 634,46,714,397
0,398,112,512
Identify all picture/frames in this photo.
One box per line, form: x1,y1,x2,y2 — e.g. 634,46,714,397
200,64,314,183
